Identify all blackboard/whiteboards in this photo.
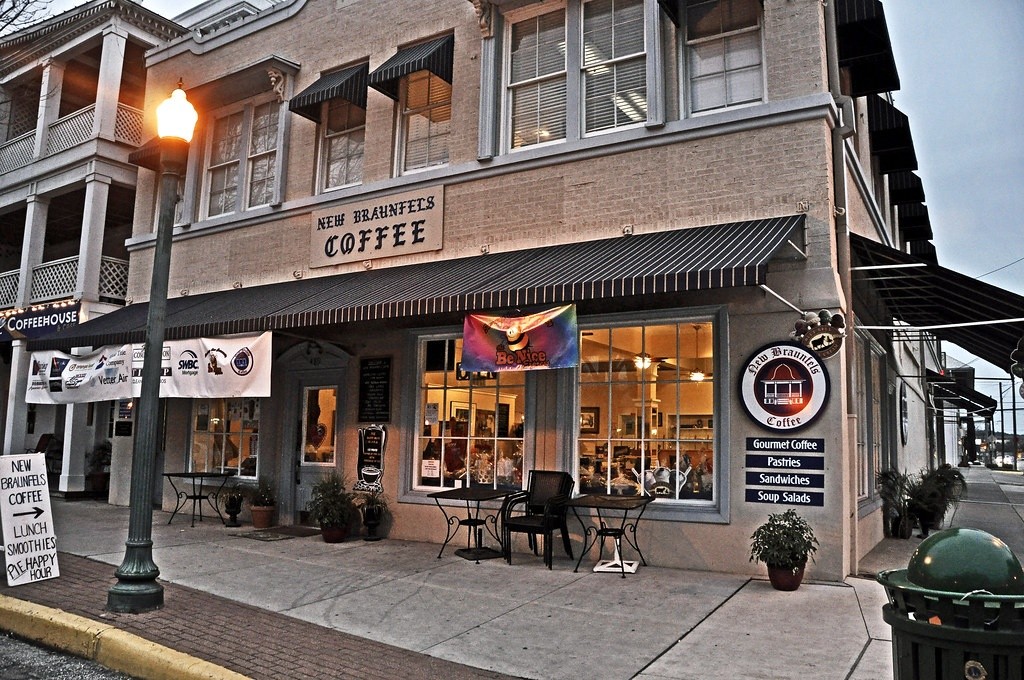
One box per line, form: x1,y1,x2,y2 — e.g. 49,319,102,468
356,354,392,425
0,452,63,588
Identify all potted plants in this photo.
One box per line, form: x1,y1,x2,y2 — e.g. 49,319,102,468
358,490,392,541
225,485,253,526
306,470,356,543
893,468,914,539
249,474,278,529
749,509,820,591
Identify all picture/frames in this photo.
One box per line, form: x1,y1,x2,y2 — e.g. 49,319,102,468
450,401,477,436
475,408,496,447
580,406,599,433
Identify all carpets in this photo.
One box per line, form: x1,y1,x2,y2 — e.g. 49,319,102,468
229,526,321,543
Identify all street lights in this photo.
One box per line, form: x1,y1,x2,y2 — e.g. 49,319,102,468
105,76,198,612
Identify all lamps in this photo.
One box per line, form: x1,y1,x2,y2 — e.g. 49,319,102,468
689,325,704,386
611,89,646,121
554,37,614,76
0,298,76,317
634,352,651,370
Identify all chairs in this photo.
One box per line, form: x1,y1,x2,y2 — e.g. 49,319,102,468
504,469,572,569
25,433,55,470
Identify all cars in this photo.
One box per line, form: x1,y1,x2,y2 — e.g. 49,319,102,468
991,454,1013,467
1016,458,1024,471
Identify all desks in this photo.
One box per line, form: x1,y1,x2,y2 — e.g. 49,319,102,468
162,472,233,528
427,486,518,564
563,493,655,578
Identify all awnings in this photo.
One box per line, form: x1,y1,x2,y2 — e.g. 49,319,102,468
926,367,997,422
834,0,900,96
868,94,918,177
367,35,452,100
889,173,938,268
288,63,370,125
850,230,1024,398
26,213,810,352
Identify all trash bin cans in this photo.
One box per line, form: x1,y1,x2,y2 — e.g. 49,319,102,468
876,527,1024,680
